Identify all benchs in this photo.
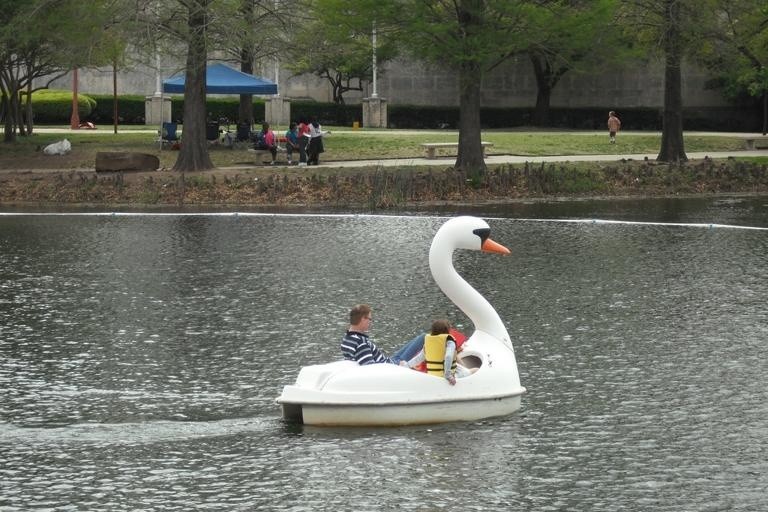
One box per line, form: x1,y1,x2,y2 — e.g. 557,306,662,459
420,141,493,159
248,148,325,167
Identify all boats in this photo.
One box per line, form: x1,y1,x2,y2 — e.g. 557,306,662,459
273,214,531,428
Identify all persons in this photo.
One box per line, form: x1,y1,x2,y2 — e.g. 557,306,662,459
608,111,621,144
257,122,278,165
285,122,301,166
341,304,426,366
307,115,324,165
297,115,311,165
400,319,480,386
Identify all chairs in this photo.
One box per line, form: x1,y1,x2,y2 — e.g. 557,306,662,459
205,121,222,144
157,122,180,152
